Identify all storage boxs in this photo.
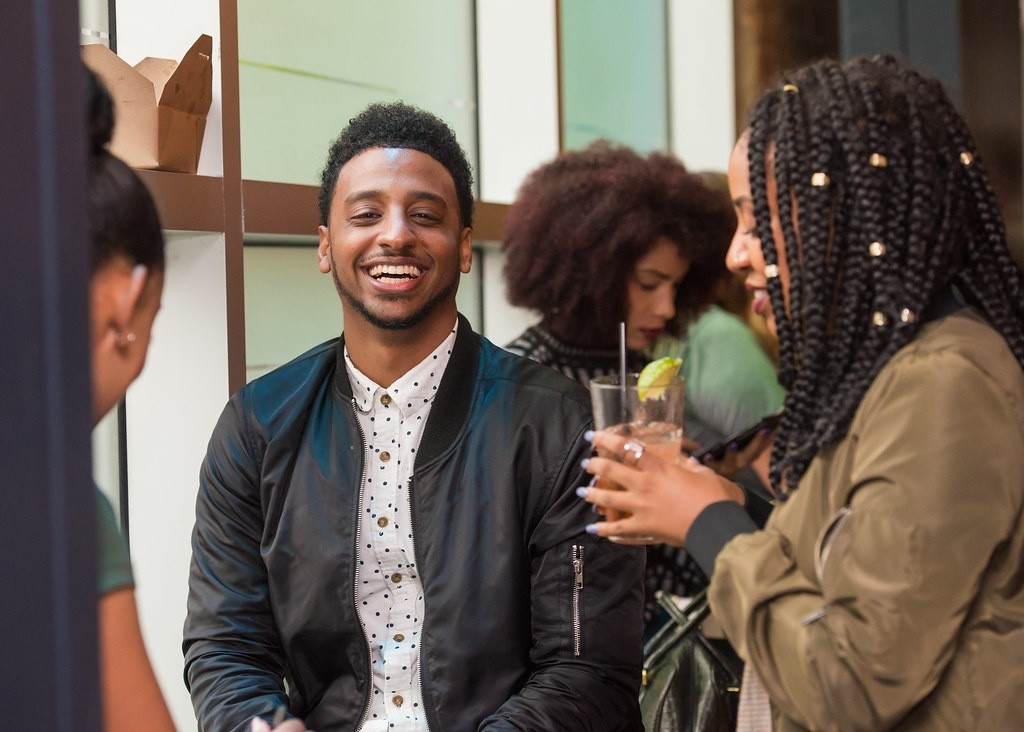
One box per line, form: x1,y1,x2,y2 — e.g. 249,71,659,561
81,33,215,174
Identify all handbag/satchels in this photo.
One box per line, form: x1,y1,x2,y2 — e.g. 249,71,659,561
637,590,742,732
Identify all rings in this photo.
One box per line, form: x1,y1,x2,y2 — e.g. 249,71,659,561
621,438,646,466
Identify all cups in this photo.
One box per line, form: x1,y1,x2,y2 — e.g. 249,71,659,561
591,374,687,545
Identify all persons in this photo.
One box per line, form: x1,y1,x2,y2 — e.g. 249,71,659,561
84,64,179,732
181,102,649,732
502,138,791,501
575,51,1024,732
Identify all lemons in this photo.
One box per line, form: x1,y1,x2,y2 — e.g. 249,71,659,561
637,356,683,403
